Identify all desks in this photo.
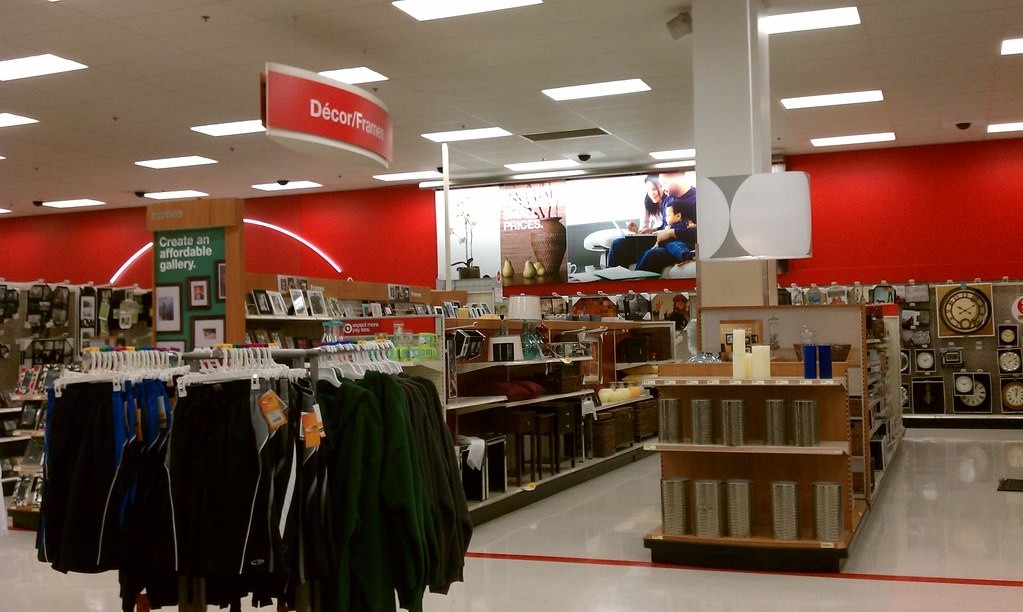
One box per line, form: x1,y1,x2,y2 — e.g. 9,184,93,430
459,400,581,502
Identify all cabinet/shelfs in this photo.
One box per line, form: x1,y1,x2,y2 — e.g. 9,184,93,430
697,305,906,512
444,317,676,517
642,362,852,556
0,396,54,528
246,273,493,358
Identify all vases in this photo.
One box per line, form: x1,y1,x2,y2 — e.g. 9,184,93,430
457,266,481,279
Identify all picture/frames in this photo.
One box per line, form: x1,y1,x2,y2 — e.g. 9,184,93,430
156,259,229,353
244,329,320,349
245,275,493,319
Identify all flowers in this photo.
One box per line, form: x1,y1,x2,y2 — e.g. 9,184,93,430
449,201,482,267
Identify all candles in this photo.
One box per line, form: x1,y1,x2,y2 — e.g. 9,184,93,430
733,330,745,380
745,353,752,380
752,346,770,380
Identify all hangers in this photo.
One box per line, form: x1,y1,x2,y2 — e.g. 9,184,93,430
54,339,402,398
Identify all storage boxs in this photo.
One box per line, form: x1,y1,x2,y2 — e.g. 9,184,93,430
590,398,660,457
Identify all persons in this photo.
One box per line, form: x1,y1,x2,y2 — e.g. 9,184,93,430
194,287,203,300
653,294,689,332
606,171,696,276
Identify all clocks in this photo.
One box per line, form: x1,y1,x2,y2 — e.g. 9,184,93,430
900,282,1023,414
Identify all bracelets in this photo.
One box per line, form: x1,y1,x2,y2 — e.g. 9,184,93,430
653,311,659,316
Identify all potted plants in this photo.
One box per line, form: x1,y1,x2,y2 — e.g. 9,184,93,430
504,180,566,274
